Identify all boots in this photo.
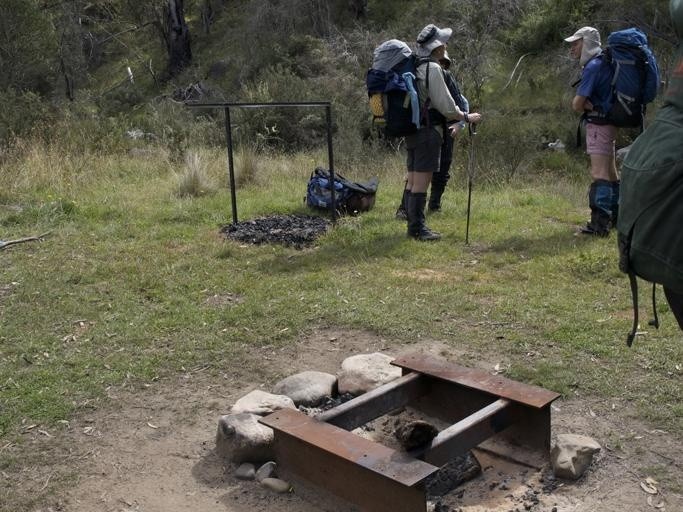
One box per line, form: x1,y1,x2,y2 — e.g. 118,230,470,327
574,179,620,238
404,189,441,241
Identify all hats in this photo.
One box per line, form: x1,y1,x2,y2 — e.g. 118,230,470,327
564,26,602,44
414,24,453,58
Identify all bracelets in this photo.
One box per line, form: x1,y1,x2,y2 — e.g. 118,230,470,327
464,112,469,123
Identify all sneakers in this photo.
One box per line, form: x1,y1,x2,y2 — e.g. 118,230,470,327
396,205,407,220
427,202,441,212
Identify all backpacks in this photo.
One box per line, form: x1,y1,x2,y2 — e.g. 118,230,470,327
583,27,659,129
303,166,380,216
367,39,445,137
617,78,683,293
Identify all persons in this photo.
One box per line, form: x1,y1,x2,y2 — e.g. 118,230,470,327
399,23,484,242
562,26,620,238
395,53,471,222
